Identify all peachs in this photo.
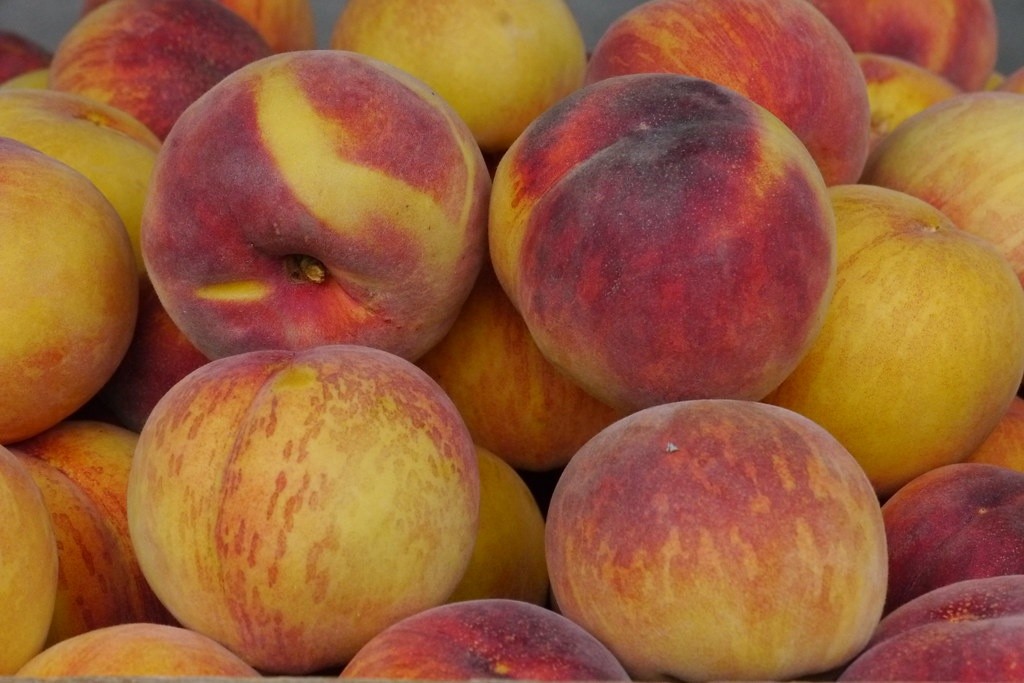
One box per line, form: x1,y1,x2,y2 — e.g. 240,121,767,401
0,0,1024,683
